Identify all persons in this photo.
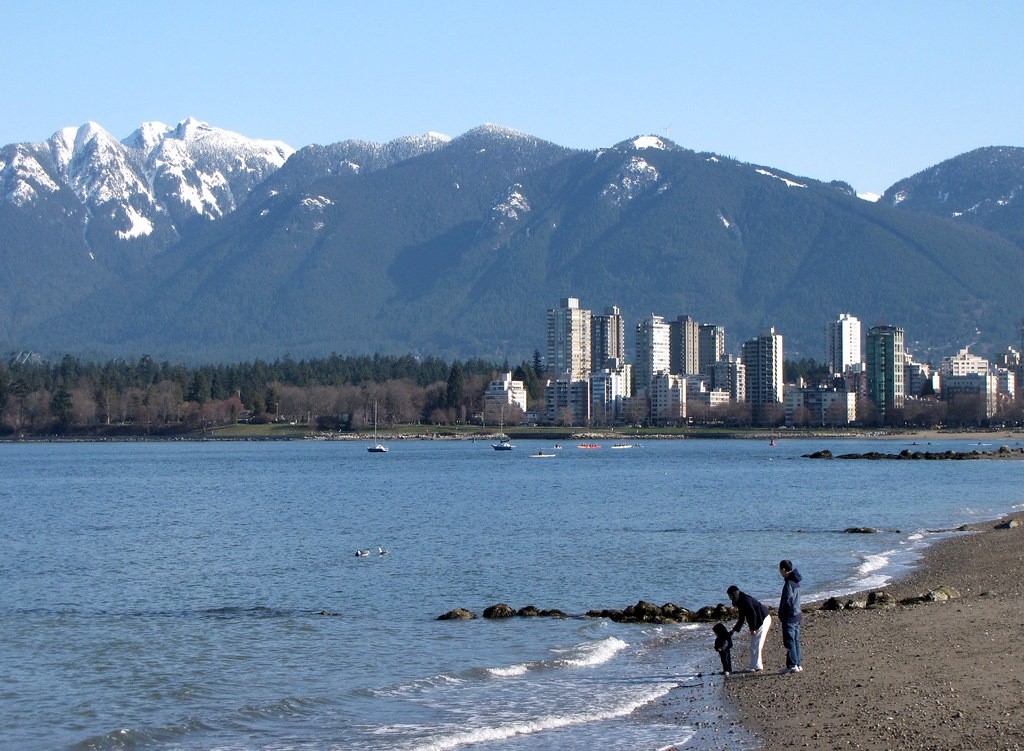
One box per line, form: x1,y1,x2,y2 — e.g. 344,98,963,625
727,586,771,672
712,624,735,675
778,560,803,673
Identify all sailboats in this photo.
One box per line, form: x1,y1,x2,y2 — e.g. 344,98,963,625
366,401,389,452
491,404,512,451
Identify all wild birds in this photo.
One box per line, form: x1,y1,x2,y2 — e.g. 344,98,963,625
378,546,390,556
355,548,371,557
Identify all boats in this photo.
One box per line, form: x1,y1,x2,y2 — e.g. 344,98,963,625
968,443,993,446
769,441,777,446
904,442,922,445
578,445,601,448
529,454,556,458
633,445,644,447
553,445,562,449
612,444,632,448
927,443,943,445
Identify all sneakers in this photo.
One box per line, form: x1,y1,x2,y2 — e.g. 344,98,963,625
784,665,802,672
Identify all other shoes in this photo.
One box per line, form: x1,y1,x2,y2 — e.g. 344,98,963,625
743,667,757,672
720,671,729,676
